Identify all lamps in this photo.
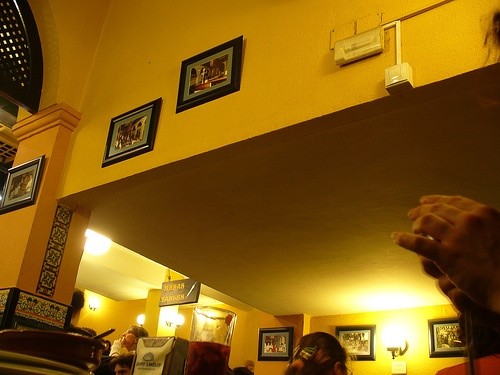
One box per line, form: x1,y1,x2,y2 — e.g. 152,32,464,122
387,342,408,359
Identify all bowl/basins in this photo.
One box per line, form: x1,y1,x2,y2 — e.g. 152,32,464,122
0,329,107,370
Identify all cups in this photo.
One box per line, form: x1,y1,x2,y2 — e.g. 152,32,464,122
182,306,237,375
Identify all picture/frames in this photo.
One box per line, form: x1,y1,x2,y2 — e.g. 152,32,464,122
429,317,467,358
175,35,244,113
102,97,162,168
257,326,294,361
335,325,378,361
0,155,45,214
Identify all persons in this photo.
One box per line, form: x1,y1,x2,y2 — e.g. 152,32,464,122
234,360,255,375
102,340,111,355
284,332,349,375
390,7,500,332
109,325,148,375
71,288,96,335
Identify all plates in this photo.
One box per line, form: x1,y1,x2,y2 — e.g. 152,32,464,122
0,347,92,375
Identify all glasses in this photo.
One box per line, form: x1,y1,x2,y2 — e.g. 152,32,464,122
127,330,138,337
